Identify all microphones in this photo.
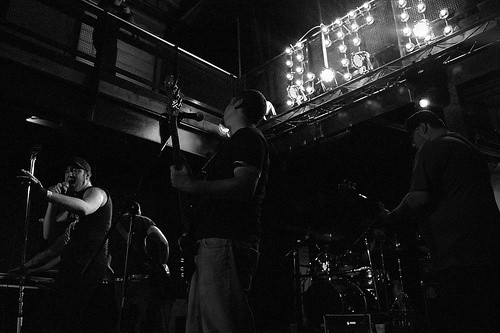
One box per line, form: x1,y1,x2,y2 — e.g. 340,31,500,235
61,182,69,195
353,229,369,246
178,112,203,120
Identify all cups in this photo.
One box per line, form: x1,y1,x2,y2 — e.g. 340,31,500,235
376,324,385,333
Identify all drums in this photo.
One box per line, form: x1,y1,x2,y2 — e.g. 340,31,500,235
303,278,368,333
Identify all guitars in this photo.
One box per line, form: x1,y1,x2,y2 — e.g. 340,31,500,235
164,72,204,295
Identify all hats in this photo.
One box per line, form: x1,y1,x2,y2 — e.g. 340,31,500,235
63,157,91,170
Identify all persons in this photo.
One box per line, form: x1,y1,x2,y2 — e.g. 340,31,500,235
170,89,270,333
373,110,500,333
16,156,169,333
